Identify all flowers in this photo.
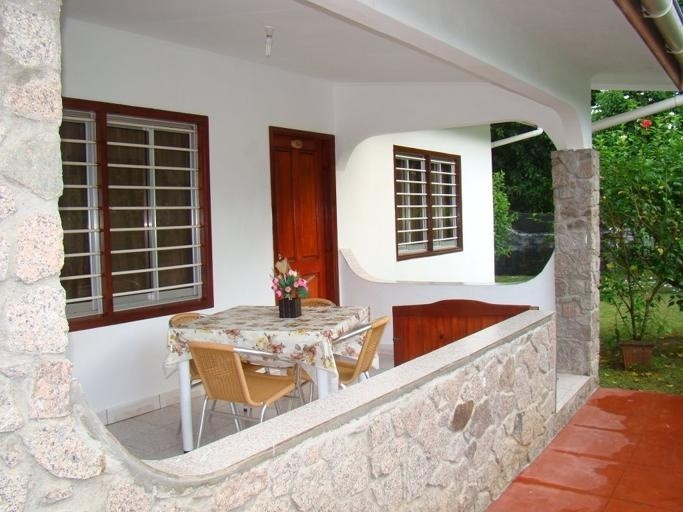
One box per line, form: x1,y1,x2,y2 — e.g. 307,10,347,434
271,268,308,298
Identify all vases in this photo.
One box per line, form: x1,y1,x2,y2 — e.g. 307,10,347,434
280,298,302,318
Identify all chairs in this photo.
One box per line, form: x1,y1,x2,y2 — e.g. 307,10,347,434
302,298,337,307
170,312,206,325
295,317,389,402
188,341,294,447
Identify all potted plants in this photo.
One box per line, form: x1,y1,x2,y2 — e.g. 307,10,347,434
604,136,681,371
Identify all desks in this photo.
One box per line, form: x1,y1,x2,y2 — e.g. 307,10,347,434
172,304,372,453
